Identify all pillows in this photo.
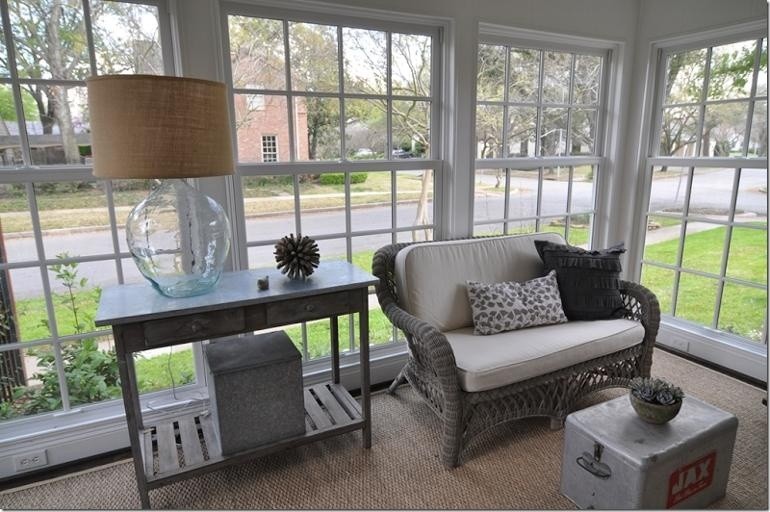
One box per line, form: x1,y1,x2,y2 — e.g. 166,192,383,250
534,239,632,322
464,267,571,334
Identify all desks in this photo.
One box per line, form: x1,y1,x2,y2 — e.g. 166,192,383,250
560,392,739,511
95,256,379,507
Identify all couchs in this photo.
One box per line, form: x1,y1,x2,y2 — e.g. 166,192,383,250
373,235,660,469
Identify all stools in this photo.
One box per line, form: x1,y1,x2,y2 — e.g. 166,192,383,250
202,329,305,455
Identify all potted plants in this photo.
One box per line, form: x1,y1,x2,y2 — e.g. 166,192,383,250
627,373,690,427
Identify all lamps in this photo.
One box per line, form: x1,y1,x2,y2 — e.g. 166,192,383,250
83,74,237,298
